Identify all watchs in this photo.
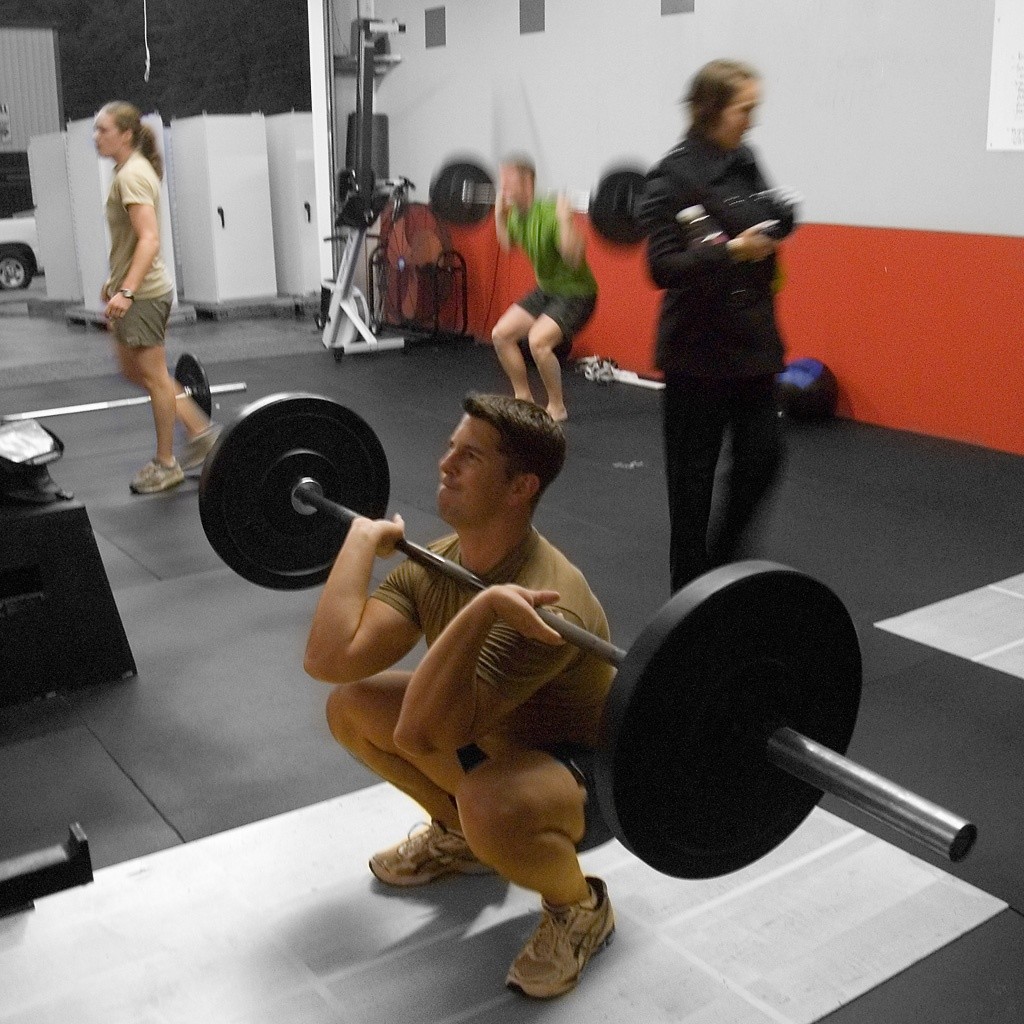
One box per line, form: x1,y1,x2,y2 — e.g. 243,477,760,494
119,287,135,302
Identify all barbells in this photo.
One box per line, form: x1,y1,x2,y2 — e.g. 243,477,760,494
198,391,976,880
1,355,248,426
430,160,649,244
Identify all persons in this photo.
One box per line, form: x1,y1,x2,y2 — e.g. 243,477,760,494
303,393,618,1003
637,58,797,597
490,148,600,420
91,98,225,494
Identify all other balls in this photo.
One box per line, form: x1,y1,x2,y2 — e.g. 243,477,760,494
773,357,837,418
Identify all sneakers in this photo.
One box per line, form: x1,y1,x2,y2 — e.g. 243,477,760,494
130,457,185,494
177,423,225,471
369,821,499,885
505,875,615,1000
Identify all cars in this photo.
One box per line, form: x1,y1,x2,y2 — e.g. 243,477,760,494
1,218,44,292
